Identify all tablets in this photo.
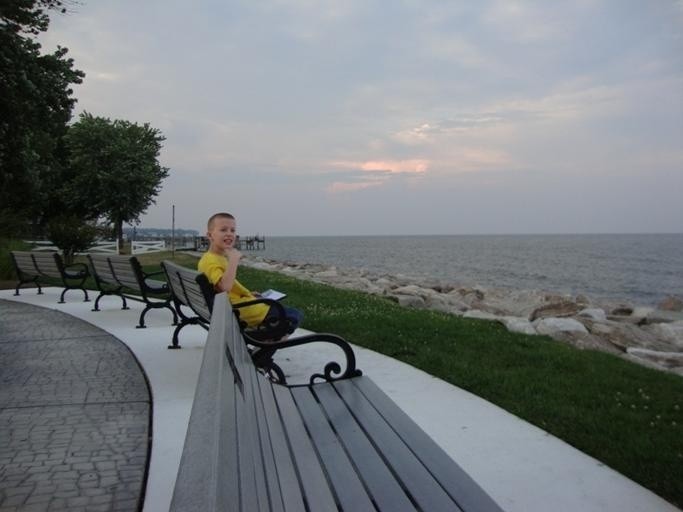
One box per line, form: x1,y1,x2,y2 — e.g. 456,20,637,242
258,289,287,305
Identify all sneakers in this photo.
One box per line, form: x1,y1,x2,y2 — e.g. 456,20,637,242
247,343,273,377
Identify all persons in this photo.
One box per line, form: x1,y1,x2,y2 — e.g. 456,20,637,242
196,212,300,366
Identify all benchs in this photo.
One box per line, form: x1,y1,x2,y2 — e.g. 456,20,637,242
155,261,298,383
164,290,507,511
6,248,89,304
85,252,180,328
